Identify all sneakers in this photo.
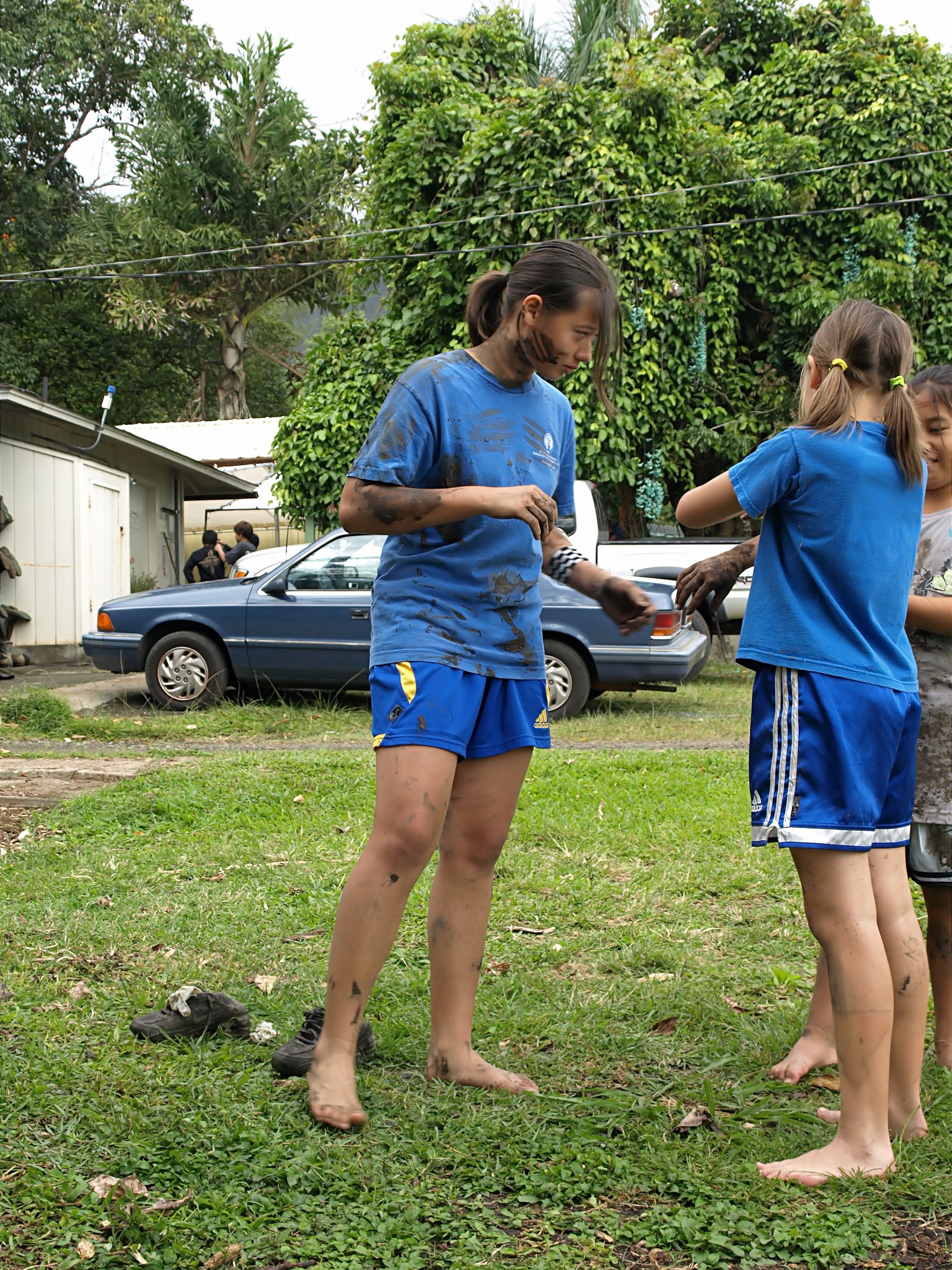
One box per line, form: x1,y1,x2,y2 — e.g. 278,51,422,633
128,985,250,1045
271,1006,376,1075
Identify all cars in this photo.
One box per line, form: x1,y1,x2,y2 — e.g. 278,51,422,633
81,525,714,727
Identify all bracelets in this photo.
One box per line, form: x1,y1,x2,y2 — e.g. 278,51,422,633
542,545,589,584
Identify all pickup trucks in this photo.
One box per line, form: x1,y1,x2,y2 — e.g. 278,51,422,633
223,477,761,634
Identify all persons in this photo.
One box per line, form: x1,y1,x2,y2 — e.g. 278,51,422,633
292,241,657,1130
183,521,259,584
676,299,929,1187
675,364,952,1086
